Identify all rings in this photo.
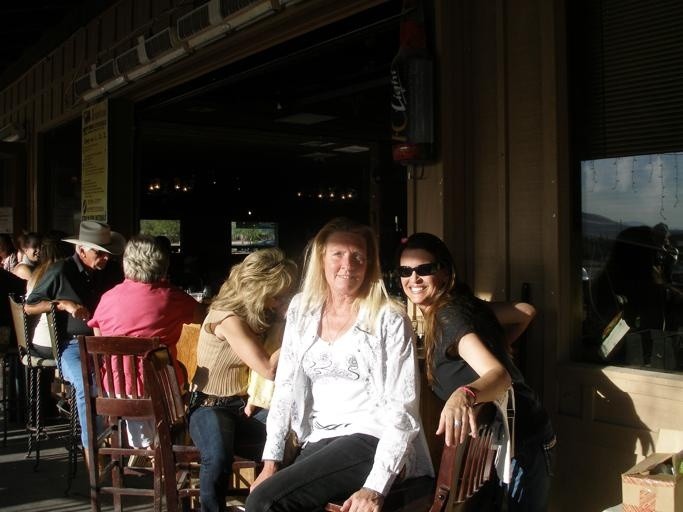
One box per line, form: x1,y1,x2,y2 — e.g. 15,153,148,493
454,419,462,428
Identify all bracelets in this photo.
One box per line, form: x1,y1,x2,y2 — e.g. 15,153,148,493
455,385,478,406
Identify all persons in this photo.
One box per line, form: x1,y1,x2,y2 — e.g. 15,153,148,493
242,217,435,512
1,219,129,482
395,232,559,511
86,233,193,448
183,244,301,512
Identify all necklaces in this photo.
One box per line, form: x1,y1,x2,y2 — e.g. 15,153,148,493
326,314,350,346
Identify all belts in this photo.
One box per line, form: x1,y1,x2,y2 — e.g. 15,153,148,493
188,392,239,407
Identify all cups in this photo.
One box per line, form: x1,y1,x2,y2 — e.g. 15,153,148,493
412,316,425,352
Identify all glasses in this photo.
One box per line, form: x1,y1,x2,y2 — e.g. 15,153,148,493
91,248,103,258
397,262,444,276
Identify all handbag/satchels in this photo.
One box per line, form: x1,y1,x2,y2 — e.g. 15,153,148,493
499,456,527,506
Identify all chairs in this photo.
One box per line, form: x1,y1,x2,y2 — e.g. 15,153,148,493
325,399,503,512
141,344,262,512
46,302,90,495
7,292,70,470
77,333,166,512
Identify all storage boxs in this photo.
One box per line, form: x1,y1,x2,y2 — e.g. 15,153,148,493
621,449,683,512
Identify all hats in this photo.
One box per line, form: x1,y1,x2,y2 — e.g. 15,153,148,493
60,220,125,255
